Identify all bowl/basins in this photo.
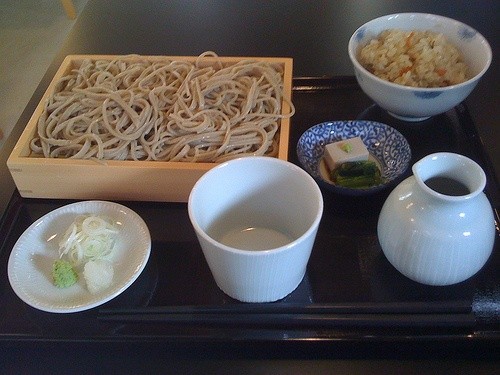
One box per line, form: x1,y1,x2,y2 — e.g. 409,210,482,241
297,120,410,205
347,12,492,121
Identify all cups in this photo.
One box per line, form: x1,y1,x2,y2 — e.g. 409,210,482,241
187,156,323,304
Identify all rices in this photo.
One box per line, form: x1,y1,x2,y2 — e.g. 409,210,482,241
358,28,468,89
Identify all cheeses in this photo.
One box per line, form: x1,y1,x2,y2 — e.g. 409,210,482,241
324,137,369,172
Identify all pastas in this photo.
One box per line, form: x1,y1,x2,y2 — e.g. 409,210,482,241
31,51,295,163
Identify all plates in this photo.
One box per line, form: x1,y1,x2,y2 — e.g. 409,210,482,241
7,200,150,312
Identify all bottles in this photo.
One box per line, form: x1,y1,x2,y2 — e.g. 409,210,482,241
377,151,495,286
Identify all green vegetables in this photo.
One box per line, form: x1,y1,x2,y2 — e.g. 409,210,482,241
330,160,382,187
